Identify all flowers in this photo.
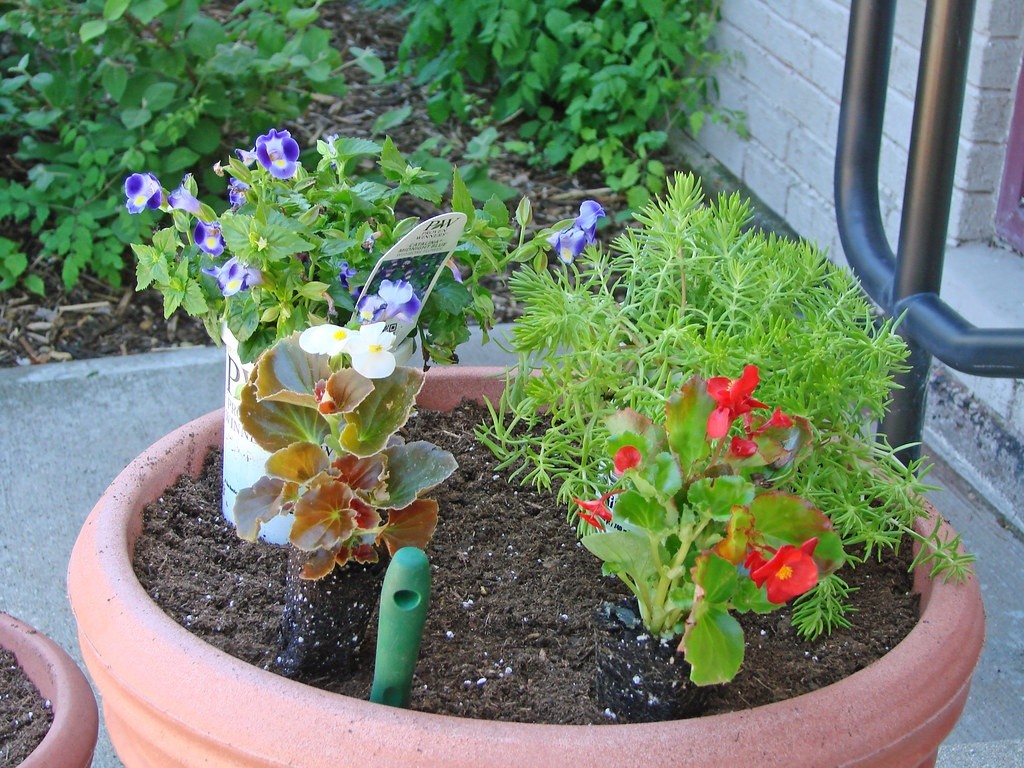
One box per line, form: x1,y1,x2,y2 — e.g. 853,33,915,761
122,129,981,721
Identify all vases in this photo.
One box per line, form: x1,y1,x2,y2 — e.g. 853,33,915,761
68,366,987,768
